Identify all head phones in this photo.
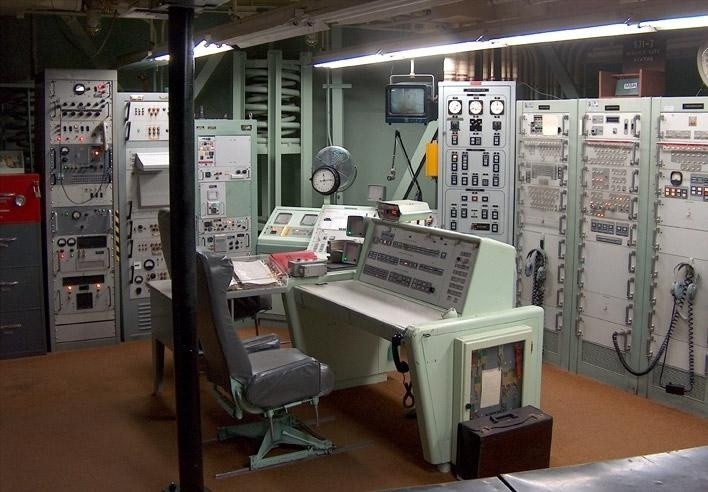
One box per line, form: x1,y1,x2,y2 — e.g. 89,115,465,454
525,247,547,281
671,260,697,300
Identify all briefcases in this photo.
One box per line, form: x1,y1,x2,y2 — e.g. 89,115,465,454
457,404,553,480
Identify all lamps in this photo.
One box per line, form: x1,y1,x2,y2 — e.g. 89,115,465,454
386,130,423,200
304,0,708,71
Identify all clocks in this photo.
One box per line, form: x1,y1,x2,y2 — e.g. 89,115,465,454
309,167,340,195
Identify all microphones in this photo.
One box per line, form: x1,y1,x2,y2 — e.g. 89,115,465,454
676,300,688,321
387,173,395,181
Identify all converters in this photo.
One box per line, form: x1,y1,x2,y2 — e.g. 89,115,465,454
666,383,684,395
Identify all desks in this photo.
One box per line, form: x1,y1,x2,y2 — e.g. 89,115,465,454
145,264,287,379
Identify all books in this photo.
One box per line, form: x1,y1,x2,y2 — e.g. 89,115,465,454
268,250,319,277
226,277,238,292
232,260,280,290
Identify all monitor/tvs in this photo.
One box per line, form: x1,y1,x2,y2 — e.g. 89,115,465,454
384,81,438,126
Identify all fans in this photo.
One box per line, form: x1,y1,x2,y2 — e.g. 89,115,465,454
312,145,357,204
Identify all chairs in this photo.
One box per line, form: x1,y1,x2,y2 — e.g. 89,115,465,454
158,209,335,479
